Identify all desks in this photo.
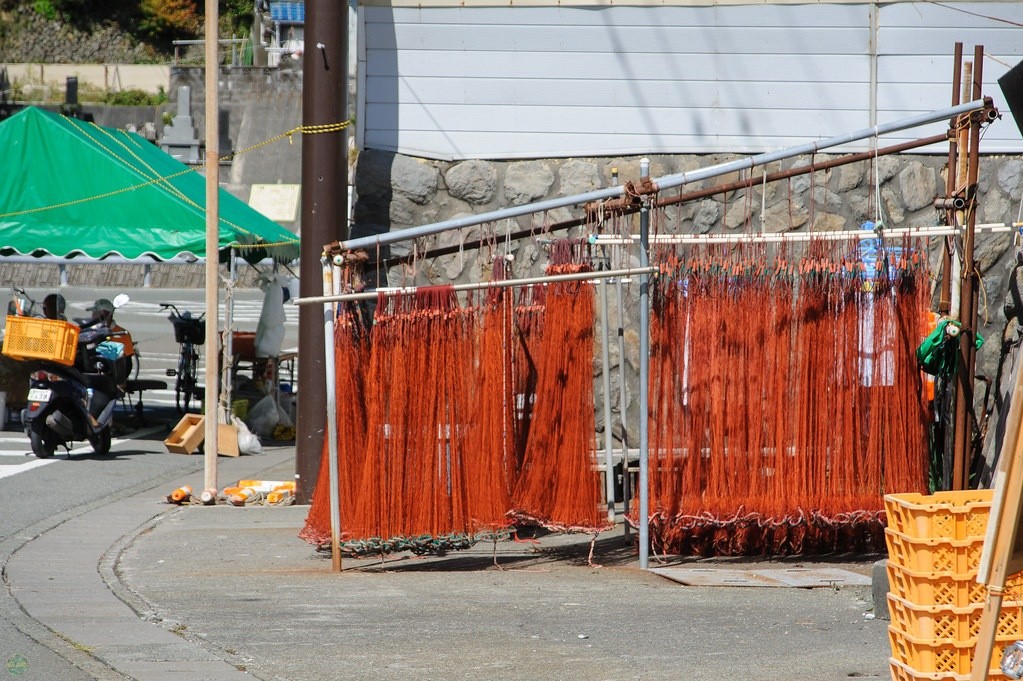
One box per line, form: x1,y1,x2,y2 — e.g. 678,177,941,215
225,353,298,411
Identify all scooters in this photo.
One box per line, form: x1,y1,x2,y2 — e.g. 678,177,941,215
0,285,142,461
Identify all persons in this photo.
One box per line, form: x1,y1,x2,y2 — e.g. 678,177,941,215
85,299,135,378
43,293,68,321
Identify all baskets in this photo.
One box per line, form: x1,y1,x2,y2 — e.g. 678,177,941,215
7,301,31,317
174,320,205,345
882,489,1023,681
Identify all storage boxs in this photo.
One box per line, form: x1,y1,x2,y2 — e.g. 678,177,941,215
873,482,1023,681
161,409,210,457
217,327,260,358
0,312,82,368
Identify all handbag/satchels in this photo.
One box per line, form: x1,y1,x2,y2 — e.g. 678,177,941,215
231,414,262,456
246,378,280,437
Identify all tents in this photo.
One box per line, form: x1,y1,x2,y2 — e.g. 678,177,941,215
0,105,300,424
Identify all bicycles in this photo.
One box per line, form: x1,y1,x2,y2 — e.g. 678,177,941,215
158,302,206,416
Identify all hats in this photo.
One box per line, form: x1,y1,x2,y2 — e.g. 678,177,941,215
85,298,115,312
